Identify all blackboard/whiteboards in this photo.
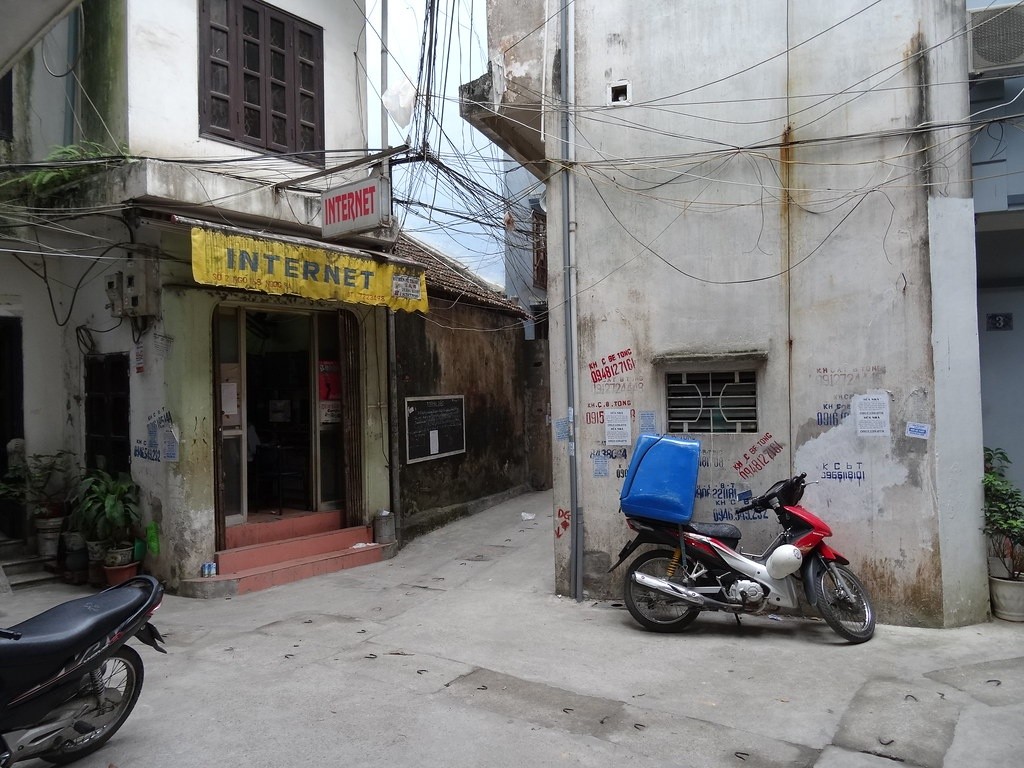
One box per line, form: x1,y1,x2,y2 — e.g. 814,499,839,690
404,395,466,465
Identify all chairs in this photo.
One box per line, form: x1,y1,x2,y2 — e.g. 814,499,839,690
254,445,310,515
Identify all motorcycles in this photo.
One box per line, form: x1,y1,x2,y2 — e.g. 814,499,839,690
607,472,875,643
0,575,168,768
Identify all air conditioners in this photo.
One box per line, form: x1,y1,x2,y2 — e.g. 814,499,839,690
966,2,1024,80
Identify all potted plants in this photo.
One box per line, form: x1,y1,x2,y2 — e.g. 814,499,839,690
0,449,160,587
978,447,1024,622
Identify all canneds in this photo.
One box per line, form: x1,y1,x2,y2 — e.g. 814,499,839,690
202,562,216,578
